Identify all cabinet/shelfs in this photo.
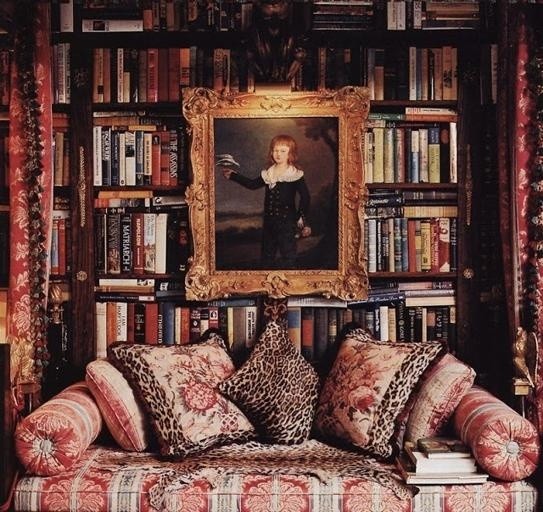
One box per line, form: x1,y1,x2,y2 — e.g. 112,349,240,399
0,2,511,412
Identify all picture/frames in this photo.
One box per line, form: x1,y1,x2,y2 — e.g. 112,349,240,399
176,79,374,312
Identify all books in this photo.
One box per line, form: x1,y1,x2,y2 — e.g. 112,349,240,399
48,110,194,276
311,2,483,100
50,2,255,104
362,107,458,273
47,280,458,364
395,436,489,485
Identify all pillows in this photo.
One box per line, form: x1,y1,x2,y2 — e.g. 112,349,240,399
217,319,322,447
83,354,155,453
405,351,478,445
106,328,262,464
310,327,450,462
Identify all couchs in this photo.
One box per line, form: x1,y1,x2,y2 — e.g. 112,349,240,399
6,327,538,512
